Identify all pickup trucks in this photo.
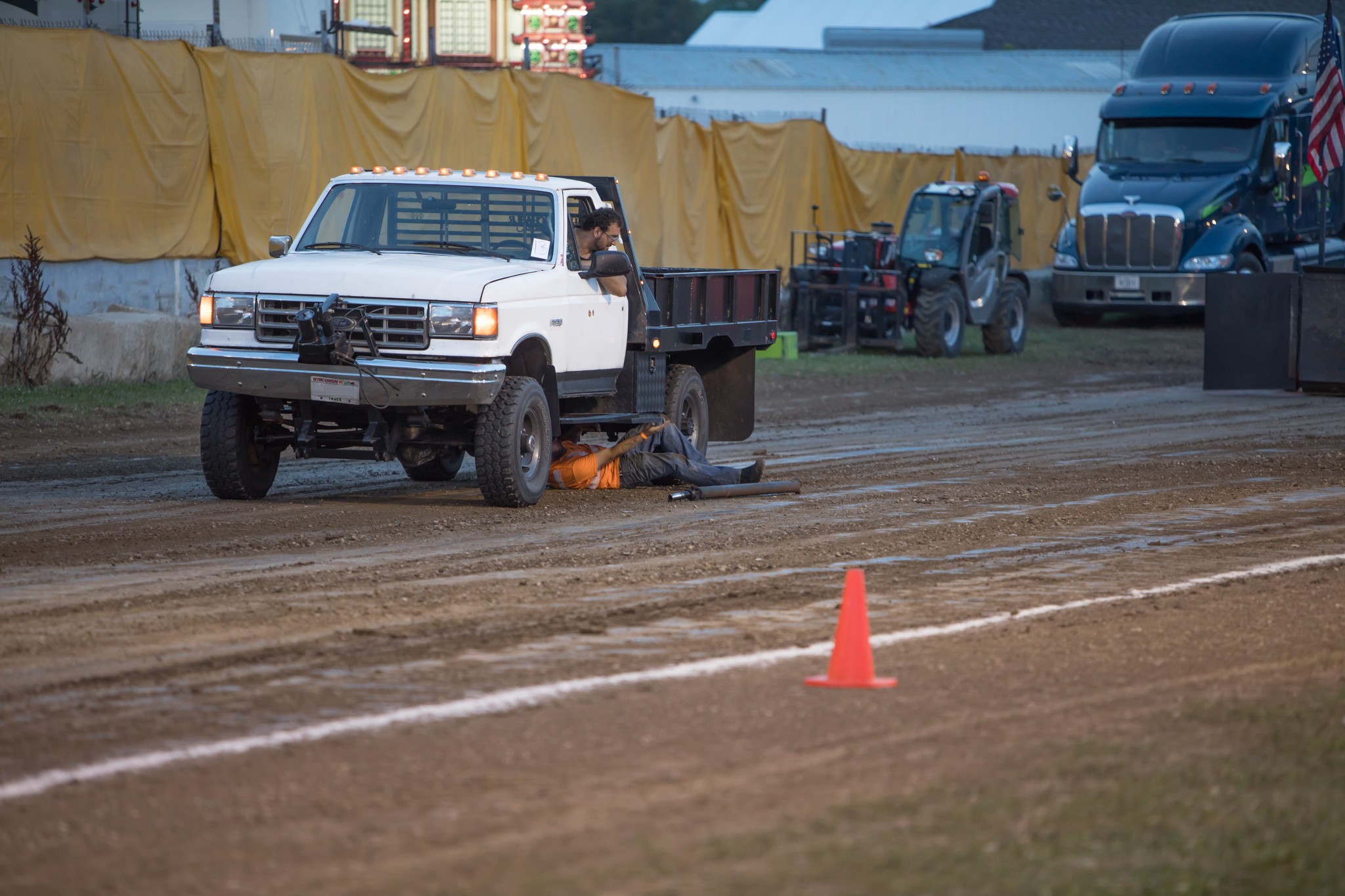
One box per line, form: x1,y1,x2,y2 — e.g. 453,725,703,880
186,166,782,506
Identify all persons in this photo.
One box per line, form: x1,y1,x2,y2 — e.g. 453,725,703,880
569,207,629,297
548,413,765,497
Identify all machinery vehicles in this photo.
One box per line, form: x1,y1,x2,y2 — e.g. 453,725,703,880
790,148,1030,357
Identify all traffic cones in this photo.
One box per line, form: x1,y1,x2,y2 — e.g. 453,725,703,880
804,569,898,690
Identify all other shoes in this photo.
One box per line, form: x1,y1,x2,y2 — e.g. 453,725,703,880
739,459,765,484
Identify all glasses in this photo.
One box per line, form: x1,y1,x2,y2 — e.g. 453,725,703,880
601,229,619,242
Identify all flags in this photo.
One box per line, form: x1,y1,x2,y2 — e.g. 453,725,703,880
1306,0,1345,183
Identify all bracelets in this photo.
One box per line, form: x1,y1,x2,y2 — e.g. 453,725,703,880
640,431,649,440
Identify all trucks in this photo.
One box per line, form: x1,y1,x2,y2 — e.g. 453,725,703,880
1047,13,1345,322
